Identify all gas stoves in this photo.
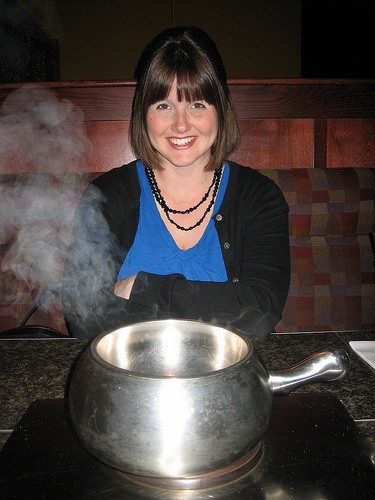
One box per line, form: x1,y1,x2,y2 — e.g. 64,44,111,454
0,392,375,500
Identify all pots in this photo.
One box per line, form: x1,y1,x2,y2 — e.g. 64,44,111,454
69,319,345,479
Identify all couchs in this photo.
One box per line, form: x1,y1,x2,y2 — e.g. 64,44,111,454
0,167,375,332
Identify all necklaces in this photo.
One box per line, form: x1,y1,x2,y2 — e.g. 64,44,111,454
143,161,223,232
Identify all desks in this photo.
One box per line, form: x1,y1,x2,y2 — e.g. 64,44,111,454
0,330,375,500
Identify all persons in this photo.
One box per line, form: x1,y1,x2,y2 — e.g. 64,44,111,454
59,34,293,343
